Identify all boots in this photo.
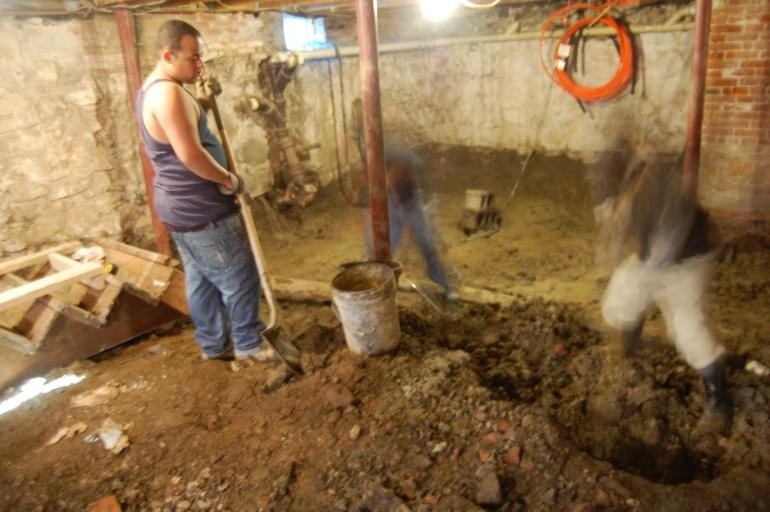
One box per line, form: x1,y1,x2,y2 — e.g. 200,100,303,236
701,365,734,439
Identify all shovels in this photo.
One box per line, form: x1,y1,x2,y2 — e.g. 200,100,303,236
203,80,305,380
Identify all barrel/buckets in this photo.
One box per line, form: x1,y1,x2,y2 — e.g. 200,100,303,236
331,264,401,358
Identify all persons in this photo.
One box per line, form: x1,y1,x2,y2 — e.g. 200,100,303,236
586,142,735,434
136,18,280,364
351,100,458,295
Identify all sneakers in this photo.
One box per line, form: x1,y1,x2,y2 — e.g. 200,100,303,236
238,347,277,363
199,348,235,361
